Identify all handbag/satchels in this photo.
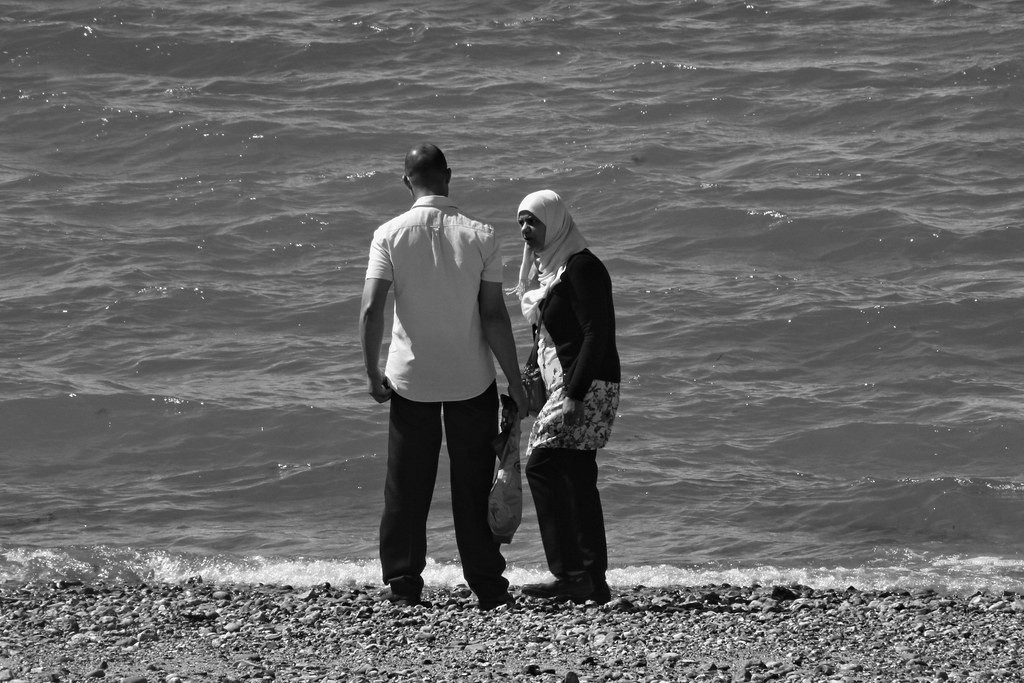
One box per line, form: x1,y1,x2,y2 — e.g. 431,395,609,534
520,365,543,413
487,404,523,536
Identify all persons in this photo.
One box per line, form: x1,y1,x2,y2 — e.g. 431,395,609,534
507,189,621,604
358,143,529,610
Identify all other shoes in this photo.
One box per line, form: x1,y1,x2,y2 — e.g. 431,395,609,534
556,580,611,602
522,579,574,597
381,590,421,604
479,592,515,610
